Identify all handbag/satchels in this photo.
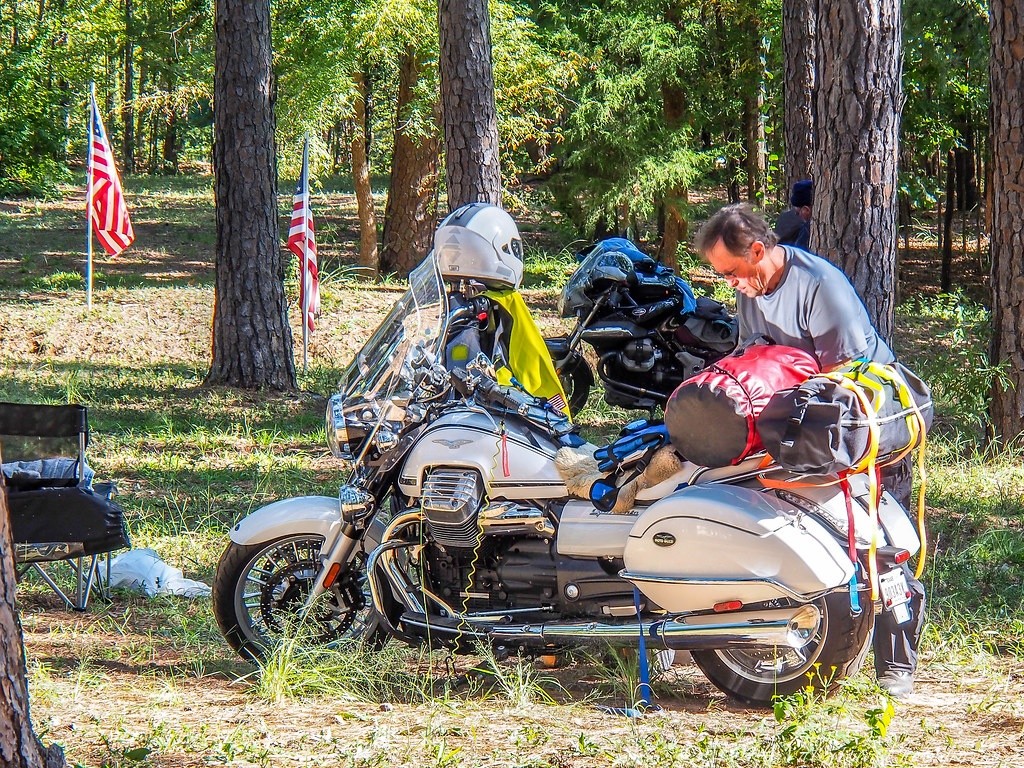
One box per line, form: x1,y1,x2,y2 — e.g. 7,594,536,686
589,420,672,513
760,357,933,475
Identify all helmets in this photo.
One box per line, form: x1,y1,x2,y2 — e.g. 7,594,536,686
434,202,524,291
592,252,634,283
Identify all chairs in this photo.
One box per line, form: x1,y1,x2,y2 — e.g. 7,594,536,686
0,403,115,611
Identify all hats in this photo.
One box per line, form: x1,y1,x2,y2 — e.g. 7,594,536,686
790,181,814,207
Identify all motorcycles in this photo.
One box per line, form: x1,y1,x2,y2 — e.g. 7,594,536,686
543,236,739,421
211,249,919,710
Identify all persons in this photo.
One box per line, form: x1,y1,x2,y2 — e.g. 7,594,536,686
789,178,816,254
691,200,929,701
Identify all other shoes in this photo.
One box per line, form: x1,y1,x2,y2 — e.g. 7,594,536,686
878,670,914,695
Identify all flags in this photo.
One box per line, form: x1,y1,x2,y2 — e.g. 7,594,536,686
85,91,137,259
285,141,321,338
